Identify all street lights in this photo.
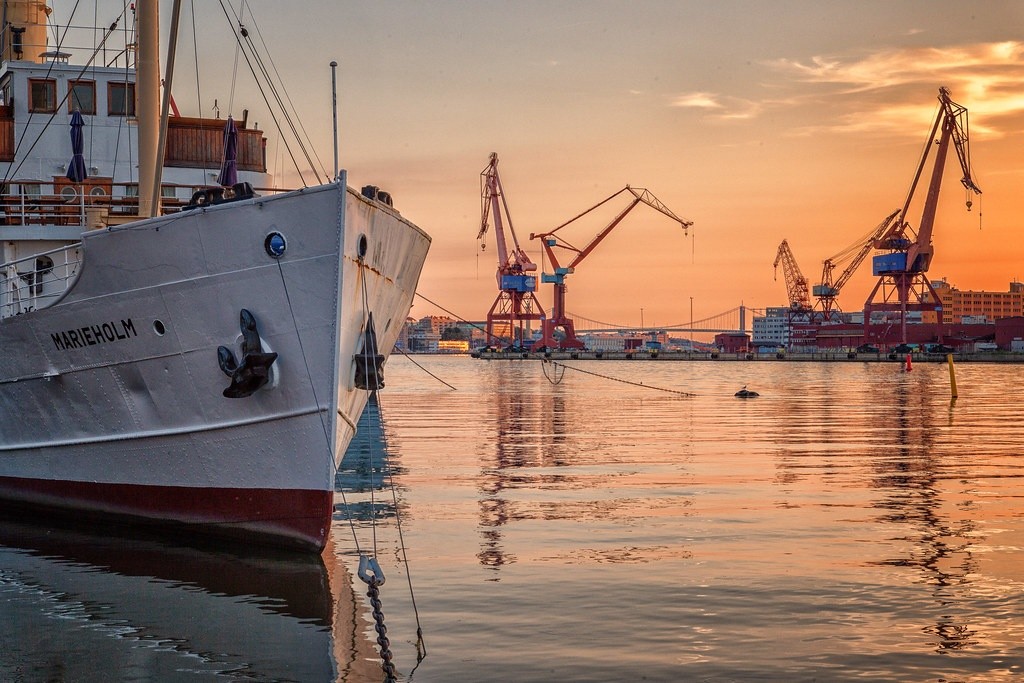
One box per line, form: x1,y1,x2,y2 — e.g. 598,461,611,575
690,297,694,351
640,308,643,334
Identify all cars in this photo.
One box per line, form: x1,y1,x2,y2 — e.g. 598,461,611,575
735,346,751,353
685,347,700,354
854,343,879,352
927,346,955,354
892,344,913,353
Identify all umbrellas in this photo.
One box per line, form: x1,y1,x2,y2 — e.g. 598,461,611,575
66,107,87,225
217,114,238,185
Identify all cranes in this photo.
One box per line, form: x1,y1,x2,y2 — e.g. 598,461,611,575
529,184,692,350
773,239,818,353
863,88,982,349
477,151,547,350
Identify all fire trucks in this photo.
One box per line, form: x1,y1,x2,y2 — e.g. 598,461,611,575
811,211,902,321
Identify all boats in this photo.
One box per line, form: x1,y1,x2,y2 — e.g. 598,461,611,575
0,1,433,563
